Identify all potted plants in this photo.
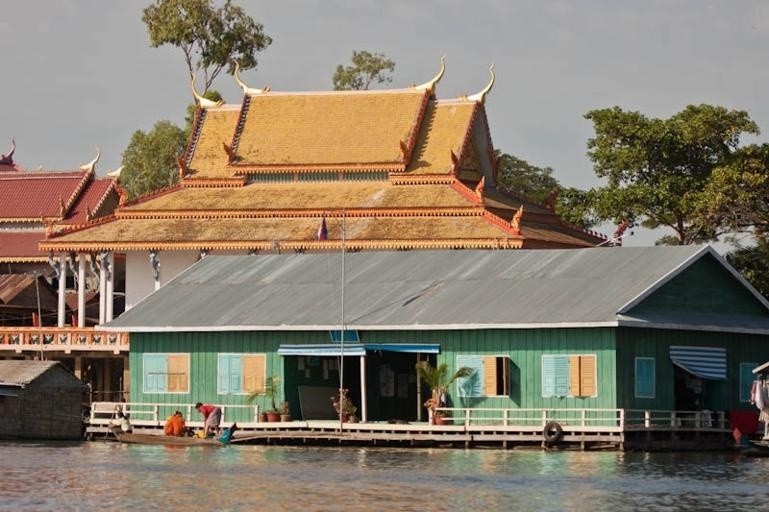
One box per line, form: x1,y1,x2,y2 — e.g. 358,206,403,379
331,388,350,422
259,376,291,422
348,404,359,424
415,361,474,424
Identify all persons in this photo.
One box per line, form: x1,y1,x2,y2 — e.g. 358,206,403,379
194,402,222,440
163,412,185,436
758,379,769,440
110,411,132,442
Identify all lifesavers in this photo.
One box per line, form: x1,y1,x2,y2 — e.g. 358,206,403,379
544,421,562,442
